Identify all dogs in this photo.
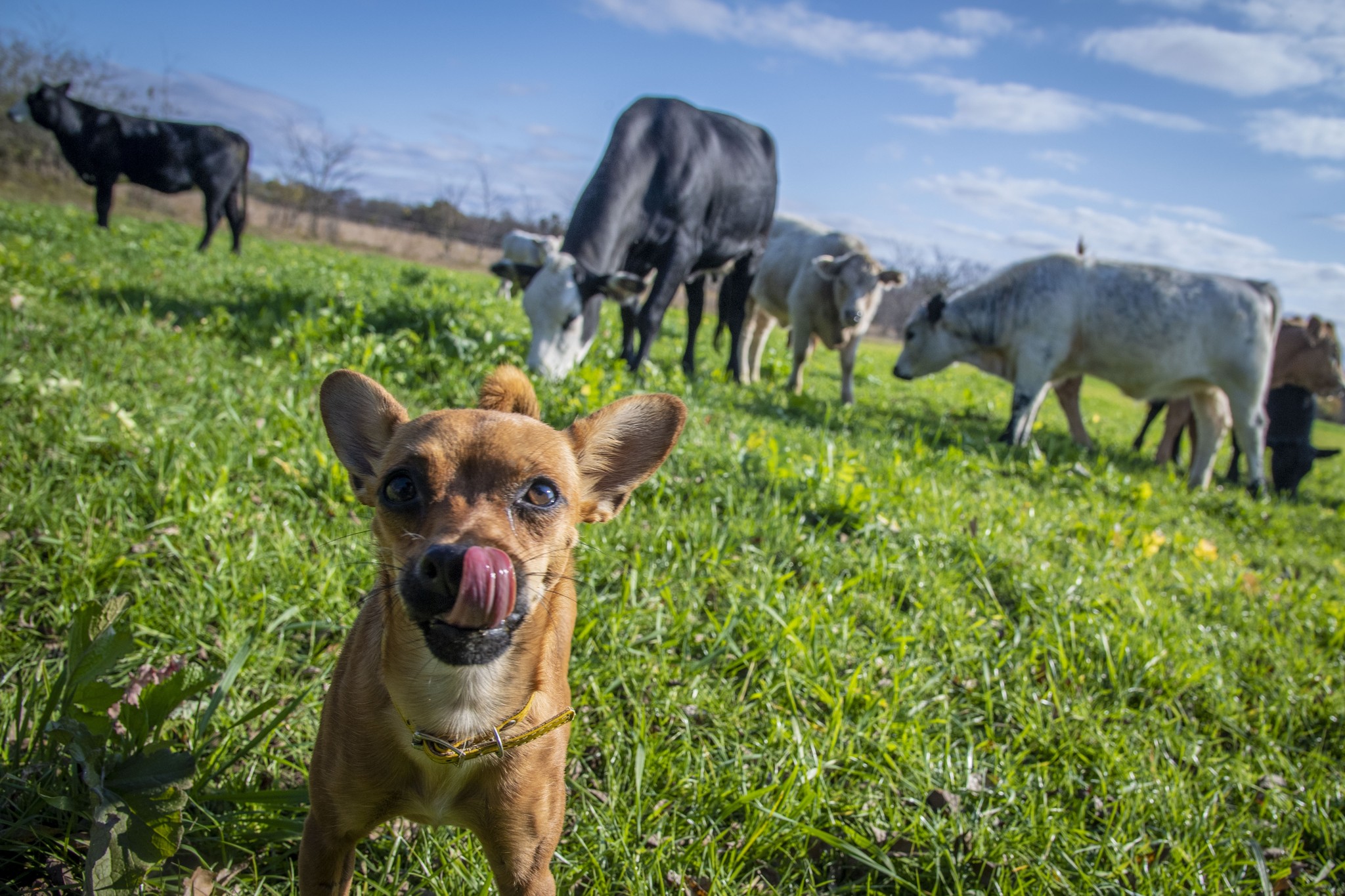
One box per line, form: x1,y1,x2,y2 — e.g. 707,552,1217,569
295,363,688,896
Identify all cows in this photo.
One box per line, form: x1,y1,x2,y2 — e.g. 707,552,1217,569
7,82,251,255
489,98,1345,504
